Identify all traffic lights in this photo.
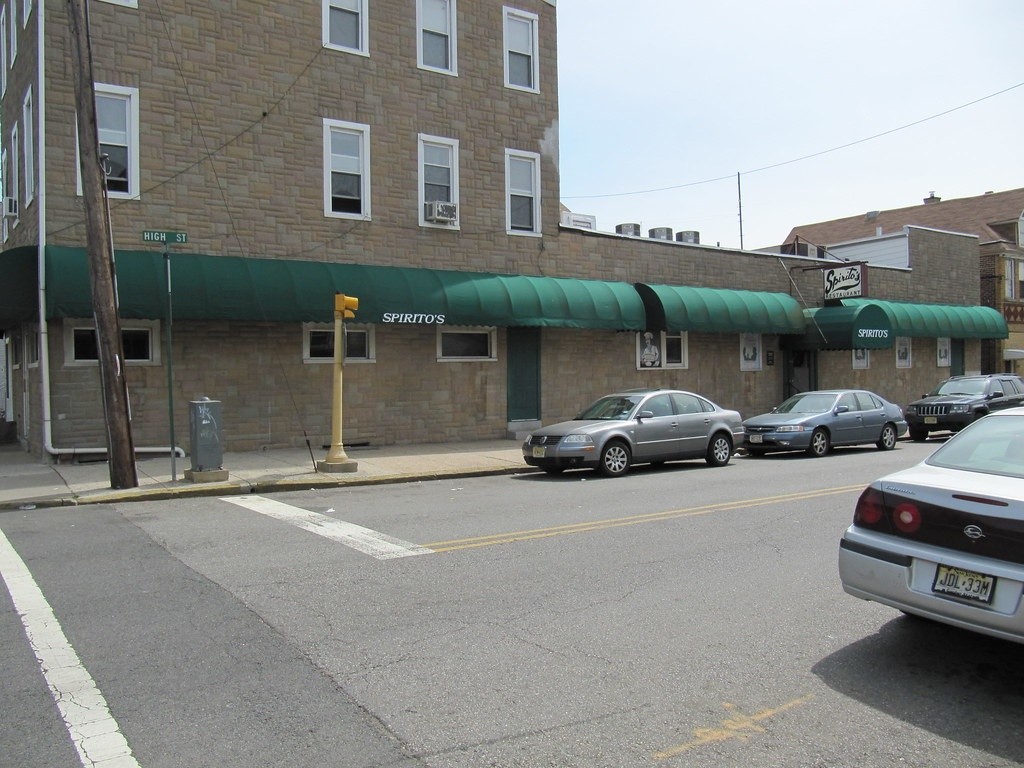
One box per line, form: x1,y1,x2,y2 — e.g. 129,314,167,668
339,294,360,321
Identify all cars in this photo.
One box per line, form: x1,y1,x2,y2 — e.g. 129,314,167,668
838,404,1024,645
743,389,911,457
522,389,746,478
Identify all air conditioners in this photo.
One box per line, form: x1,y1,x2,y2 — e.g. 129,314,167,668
427,200,457,221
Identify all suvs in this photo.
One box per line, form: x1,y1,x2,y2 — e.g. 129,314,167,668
905,372,1024,441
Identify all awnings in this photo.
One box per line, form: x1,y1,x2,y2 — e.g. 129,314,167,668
783,305,893,352
634,283,806,333
833,298,1009,340
0,245,39,336
44,245,647,331
